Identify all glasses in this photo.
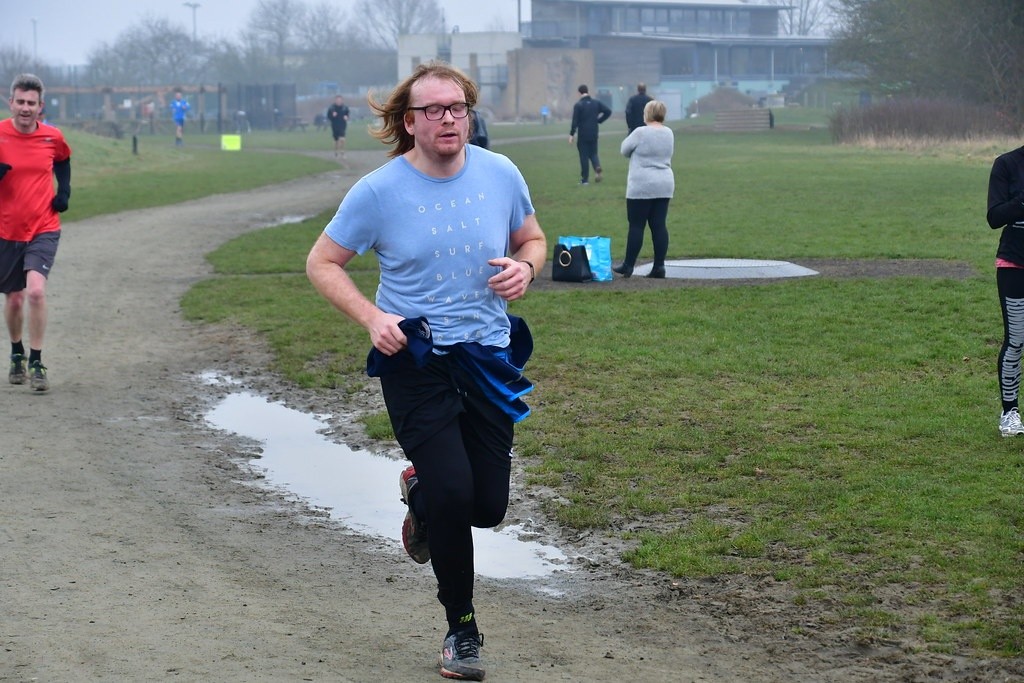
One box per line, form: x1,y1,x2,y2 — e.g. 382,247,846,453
406,102,471,122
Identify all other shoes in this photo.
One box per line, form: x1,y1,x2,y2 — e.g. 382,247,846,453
577,177,588,185
594,167,603,184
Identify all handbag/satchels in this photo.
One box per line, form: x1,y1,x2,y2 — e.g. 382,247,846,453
552,244,592,283
557,235,613,282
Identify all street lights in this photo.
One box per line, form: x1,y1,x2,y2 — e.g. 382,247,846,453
183,2,202,45
31,18,40,67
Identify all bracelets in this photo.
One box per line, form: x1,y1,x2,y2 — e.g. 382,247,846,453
519,260,535,285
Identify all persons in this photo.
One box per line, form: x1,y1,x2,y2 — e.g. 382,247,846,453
541,104,548,124
986,146,1024,439
626,82,654,136
0,72,74,393
468,107,489,150
169,88,190,144
327,94,350,159
568,85,612,185
306,56,548,681
613,99,675,279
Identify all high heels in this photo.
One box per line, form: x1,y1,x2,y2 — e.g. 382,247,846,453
613,263,633,278
647,263,665,278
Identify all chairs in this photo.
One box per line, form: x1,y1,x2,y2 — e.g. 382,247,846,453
289,116,309,133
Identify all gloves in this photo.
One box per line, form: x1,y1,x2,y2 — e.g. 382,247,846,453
0,164,12,179
52,193,69,212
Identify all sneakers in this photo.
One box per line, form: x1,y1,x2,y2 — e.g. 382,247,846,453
998,406,1024,438
27,359,50,394
399,465,432,564
9,354,26,384
437,629,485,681
